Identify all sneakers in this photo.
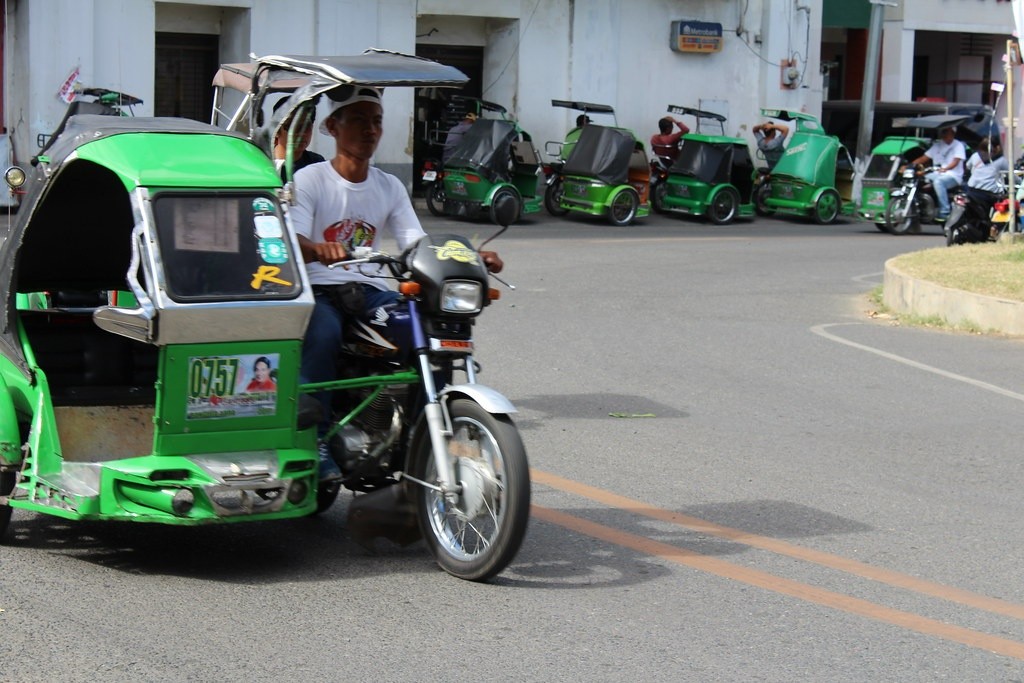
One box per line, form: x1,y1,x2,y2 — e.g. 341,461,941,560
318,444,344,483
934,216,947,222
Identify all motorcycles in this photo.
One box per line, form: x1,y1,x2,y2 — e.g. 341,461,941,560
540,98,659,226
856,114,982,237
750,109,859,224
944,155,1024,246
421,95,546,226
0,46,532,583
648,104,758,226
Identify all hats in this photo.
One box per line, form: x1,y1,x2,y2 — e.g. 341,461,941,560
576,115,594,127
465,113,479,121
318,88,384,137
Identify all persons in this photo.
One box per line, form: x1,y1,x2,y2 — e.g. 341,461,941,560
752,121,789,170
651,116,690,171
562,115,594,163
259,93,326,184
245,357,277,393
898,124,966,222
284,83,504,482
965,136,1022,238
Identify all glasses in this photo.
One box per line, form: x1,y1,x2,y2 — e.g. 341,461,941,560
281,122,314,131
